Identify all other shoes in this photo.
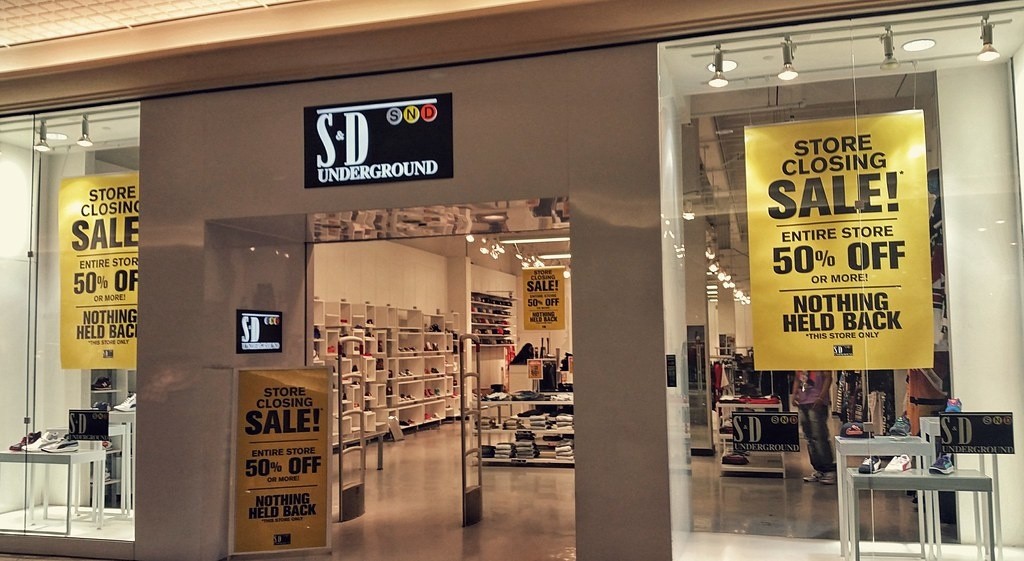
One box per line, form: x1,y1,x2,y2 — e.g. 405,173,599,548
472,295,514,346
91,402,110,411
803,471,820,483
821,472,836,484
312,313,458,427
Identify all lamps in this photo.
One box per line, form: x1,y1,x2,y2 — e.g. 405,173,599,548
682,199,695,222
33,120,51,152
706,42,728,88
77,113,93,147
978,10,1001,61
879,25,901,71
778,33,798,83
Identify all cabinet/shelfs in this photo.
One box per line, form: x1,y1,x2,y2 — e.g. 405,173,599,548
314,297,466,448
470,393,575,464
716,394,786,479
470,291,517,348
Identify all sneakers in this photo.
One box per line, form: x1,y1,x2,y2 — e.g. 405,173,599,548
41,435,78,453
113,393,136,411
928,455,954,475
889,415,911,436
90,472,111,484
858,456,882,474
90,376,111,389
945,398,962,412
21,432,59,452
885,454,911,472
10,431,41,451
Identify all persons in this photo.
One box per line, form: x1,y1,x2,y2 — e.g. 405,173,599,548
510,343,534,365
792,370,835,484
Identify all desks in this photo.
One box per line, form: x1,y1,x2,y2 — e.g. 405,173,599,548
834,434,934,559
0,444,107,536
846,465,995,561
